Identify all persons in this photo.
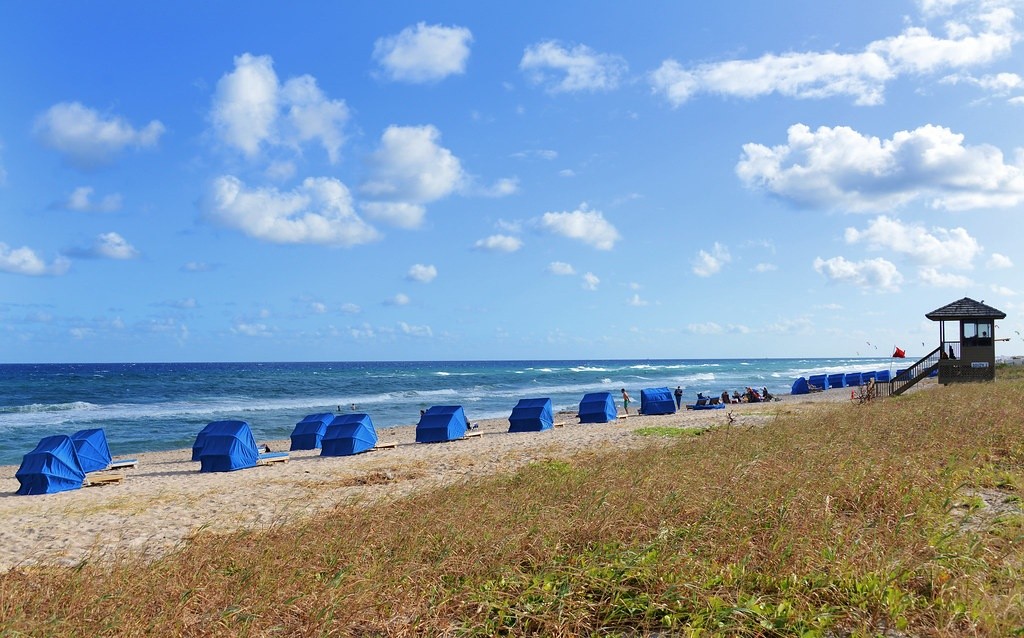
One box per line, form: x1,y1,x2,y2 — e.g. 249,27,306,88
338,403,356,411
675,386,682,409
982,331,988,337
420,410,424,416
621,389,631,414
699,386,772,405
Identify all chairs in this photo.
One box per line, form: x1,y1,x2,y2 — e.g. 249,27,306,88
507,397,564,433
14,413,399,495
686,398,707,411
637,386,676,416
415,405,482,444
790,367,938,395
578,391,628,424
709,397,719,405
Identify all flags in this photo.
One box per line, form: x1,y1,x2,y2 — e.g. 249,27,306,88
892,347,906,358
